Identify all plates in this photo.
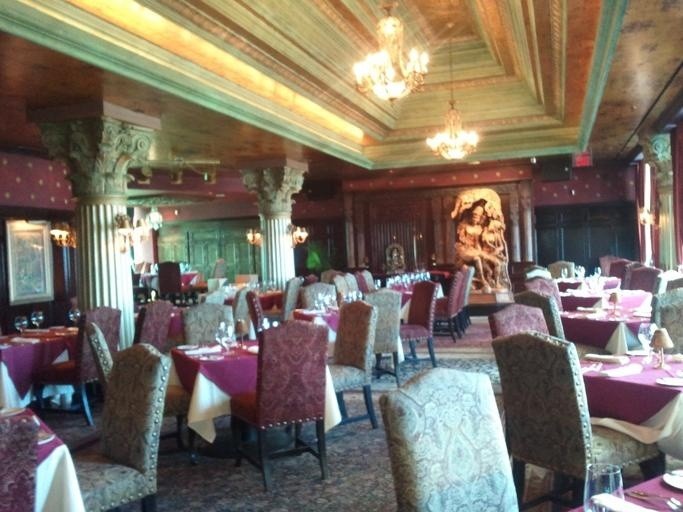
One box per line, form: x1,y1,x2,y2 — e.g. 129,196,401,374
656,376,683,386
200,356,224,361
662,469,683,488
1,407,25,417
177,345,200,350
36,432,55,445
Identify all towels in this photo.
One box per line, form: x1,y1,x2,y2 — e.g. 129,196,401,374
585,353,629,365
576,306,599,312
184,348,220,355
11,336,40,344
590,492,658,512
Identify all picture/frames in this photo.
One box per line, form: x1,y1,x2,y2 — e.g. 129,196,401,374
6,218,56,307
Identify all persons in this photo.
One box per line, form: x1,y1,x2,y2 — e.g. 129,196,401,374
454,205,506,295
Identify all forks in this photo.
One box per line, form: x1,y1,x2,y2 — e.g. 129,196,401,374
625,491,679,511
632,489,682,507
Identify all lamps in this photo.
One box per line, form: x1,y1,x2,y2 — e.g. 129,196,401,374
245,228,261,247
50,222,75,249
291,225,308,247
351,1,430,110
235,318,247,350
651,328,674,370
639,206,657,227
424,23,482,162
116,215,151,250
599,255,621,276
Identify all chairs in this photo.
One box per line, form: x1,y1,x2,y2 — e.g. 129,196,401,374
609,292,653,316
514,290,565,341
434,272,462,343
247,291,267,331
133,300,171,347
333,275,349,303
399,281,439,368
443,266,476,326
620,261,646,289
652,269,683,296
651,287,683,354
523,277,563,312
546,261,575,281
300,281,338,307
665,276,683,293
491,330,665,511
363,270,376,293
33,307,122,429
437,265,468,334
229,320,330,493
157,261,190,307
609,258,631,279
84,321,200,466
344,272,359,295
322,270,335,286
0,417,36,512
181,303,235,343
362,288,402,389
233,285,253,341
264,278,305,323
524,268,553,282
305,274,318,287
624,267,663,294
206,289,225,305
323,299,379,429
194,259,226,291
354,271,369,294
67,343,173,511
379,367,519,512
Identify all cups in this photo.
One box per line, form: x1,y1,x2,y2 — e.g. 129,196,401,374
15,317,27,346
584,464,624,512
70,309,80,336
561,267,567,279
638,322,659,364
595,266,601,281
374,279,381,290
215,321,236,356
30,311,43,339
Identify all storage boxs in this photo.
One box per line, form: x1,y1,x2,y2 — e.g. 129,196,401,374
488,302,550,337
